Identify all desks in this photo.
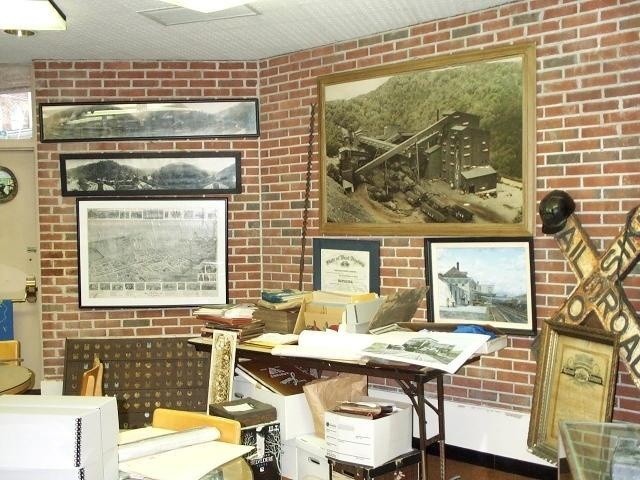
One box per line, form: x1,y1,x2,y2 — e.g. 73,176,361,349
187,338,481,480
118,428,254,480
0,363,35,396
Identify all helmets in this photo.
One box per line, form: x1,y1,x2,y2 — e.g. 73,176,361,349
539,191,575,234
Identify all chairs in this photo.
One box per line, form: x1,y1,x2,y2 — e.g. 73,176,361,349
153,407,242,480
82,355,103,397
0,340,21,364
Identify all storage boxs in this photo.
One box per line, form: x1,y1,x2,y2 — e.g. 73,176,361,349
232,378,315,440
323,400,413,466
329,452,424,480
297,449,332,480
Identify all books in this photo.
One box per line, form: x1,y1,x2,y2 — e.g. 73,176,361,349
192,288,508,373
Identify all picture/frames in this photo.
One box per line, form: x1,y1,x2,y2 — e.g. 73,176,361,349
526,319,621,467
313,238,380,297
0,166,18,203
39,98,259,142
76,197,228,309
60,151,241,196
316,41,537,237
424,238,536,335
